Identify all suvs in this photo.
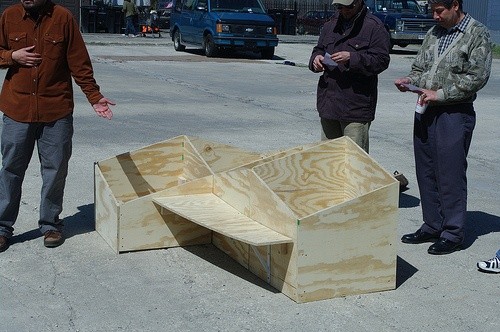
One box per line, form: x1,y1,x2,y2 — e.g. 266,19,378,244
363,0,438,49
169,0,280,59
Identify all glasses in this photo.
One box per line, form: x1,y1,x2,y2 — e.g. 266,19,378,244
335,0,358,9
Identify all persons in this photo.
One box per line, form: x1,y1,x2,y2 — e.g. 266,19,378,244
121,0,141,37
150,0,161,29
394,1,492,256
308,0,392,155
477,248,500,274
0,0,117,252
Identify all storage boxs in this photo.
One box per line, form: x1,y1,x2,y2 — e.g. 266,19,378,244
94,133,400,303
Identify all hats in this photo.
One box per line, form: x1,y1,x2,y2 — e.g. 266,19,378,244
331,0,353,5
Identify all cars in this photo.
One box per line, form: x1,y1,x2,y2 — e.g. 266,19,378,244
156,9,173,28
296,9,337,36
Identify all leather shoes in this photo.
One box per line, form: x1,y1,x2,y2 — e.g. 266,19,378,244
401,229,438,243
428,238,459,254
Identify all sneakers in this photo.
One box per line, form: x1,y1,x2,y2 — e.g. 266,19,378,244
477,256,500,273
44,231,64,246
0,235,10,251
